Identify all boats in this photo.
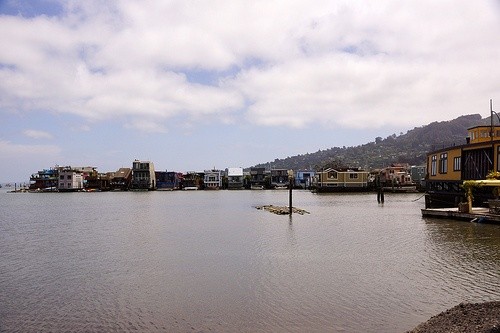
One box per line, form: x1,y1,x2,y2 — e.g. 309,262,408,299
156,167,314,191
375,163,427,192
